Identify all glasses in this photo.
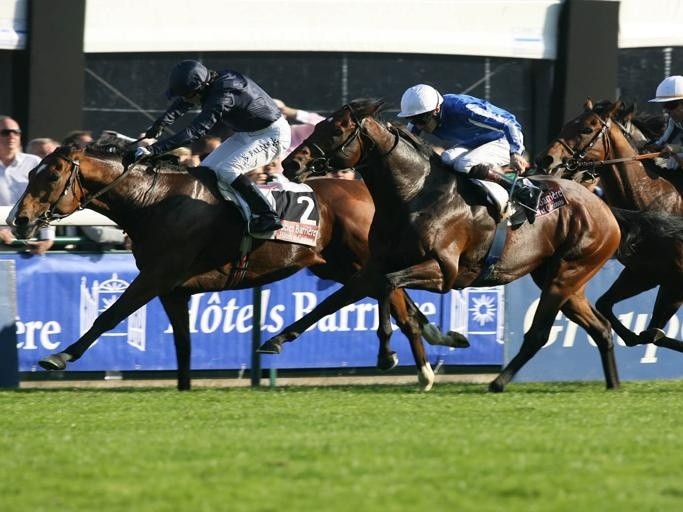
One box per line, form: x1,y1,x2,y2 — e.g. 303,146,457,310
408,112,433,127
0,128,21,136
660,101,683,109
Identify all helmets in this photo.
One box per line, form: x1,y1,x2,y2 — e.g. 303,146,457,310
645,74,683,104
162,57,211,100
394,83,444,119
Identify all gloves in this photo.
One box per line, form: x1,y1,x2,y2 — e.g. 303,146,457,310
508,154,529,176
132,146,151,161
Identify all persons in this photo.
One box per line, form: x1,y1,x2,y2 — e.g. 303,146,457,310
645,74,683,154
132,58,292,233
0,97,355,255
397,84,533,200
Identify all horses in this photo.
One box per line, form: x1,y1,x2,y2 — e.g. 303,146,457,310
254,96,624,396
616,100,682,200
543,98,683,354
5,128,471,395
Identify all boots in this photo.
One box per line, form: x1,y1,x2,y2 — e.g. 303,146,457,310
485,167,542,226
229,173,284,237
